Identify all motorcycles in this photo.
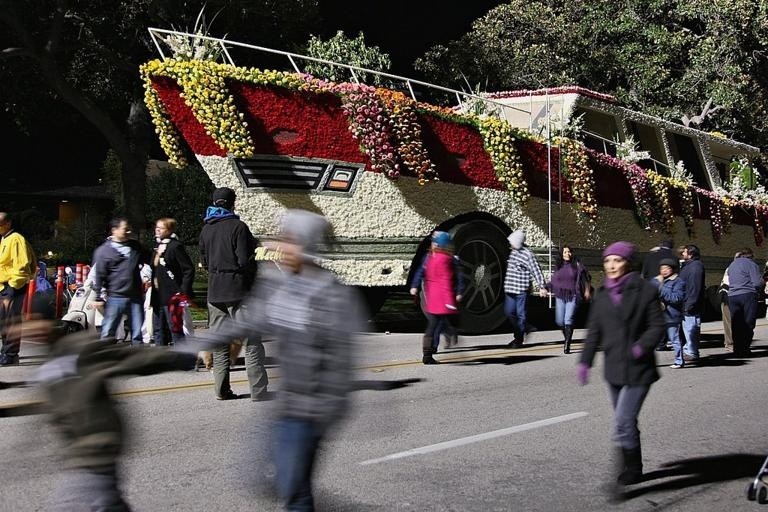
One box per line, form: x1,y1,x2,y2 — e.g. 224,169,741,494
60,266,128,345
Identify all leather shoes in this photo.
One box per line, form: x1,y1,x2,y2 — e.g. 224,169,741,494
422,357,442,364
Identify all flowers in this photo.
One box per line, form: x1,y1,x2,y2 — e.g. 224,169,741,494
139,57,766,287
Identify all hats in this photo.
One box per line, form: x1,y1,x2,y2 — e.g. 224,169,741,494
280,207,331,250
657,258,679,275
506,229,527,251
213,186,236,208
603,240,637,264
429,229,451,251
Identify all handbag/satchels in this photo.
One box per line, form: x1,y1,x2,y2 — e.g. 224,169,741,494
181,302,195,338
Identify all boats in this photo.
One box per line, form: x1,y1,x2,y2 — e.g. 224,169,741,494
140,26,768,335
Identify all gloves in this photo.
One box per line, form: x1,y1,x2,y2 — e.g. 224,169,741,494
576,363,590,387
631,343,644,360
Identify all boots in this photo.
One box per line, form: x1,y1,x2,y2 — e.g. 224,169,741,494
605,444,645,495
560,325,565,338
564,324,574,354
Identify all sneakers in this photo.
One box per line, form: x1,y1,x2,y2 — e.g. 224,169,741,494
0,351,20,366
506,338,524,347
669,353,701,370
215,390,276,402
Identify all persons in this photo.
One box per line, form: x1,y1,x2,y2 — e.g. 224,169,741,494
410,230,464,349
422,232,458,365
540,245,592,353
575,241,667,501
504,230,548,348
641,239,768,368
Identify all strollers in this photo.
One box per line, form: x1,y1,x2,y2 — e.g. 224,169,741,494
747,446,768,506
